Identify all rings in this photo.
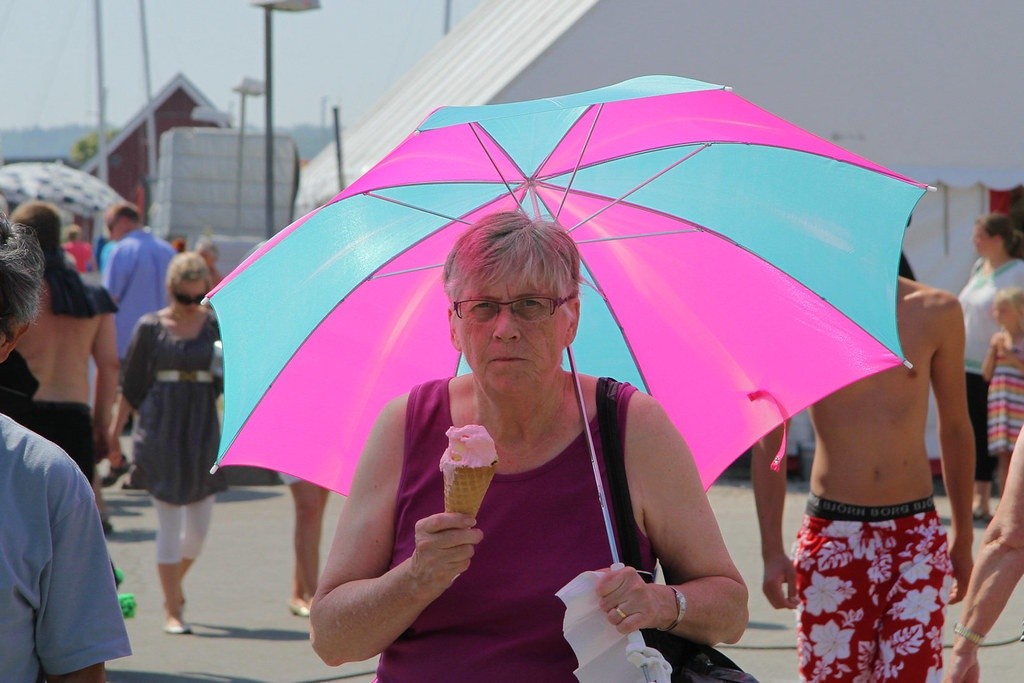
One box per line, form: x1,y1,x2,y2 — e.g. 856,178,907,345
615,607,626,619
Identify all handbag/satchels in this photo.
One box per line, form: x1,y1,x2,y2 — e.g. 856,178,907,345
596,376,757,683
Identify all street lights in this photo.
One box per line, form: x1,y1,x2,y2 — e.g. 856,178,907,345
228,73,268,238
253,0,323,238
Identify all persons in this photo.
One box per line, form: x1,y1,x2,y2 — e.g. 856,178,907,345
0,204,331,683
308,211,749,683
751,212,1024,683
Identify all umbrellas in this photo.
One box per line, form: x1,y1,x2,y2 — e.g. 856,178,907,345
200,75,936,574
0,160,128,217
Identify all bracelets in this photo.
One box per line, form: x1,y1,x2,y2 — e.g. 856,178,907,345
657,585,686,632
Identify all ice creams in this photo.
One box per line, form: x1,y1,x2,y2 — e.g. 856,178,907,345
439,425,498,529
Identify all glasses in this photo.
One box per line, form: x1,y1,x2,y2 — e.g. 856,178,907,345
107,217,122,232
453,295,575,321
171,289,207,307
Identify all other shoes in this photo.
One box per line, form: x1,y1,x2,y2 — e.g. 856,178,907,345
289,597,310,618
100,455,131,488
972,511,993,528
164,617,191,634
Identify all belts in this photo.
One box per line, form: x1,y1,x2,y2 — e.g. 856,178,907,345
154,369,214,384
